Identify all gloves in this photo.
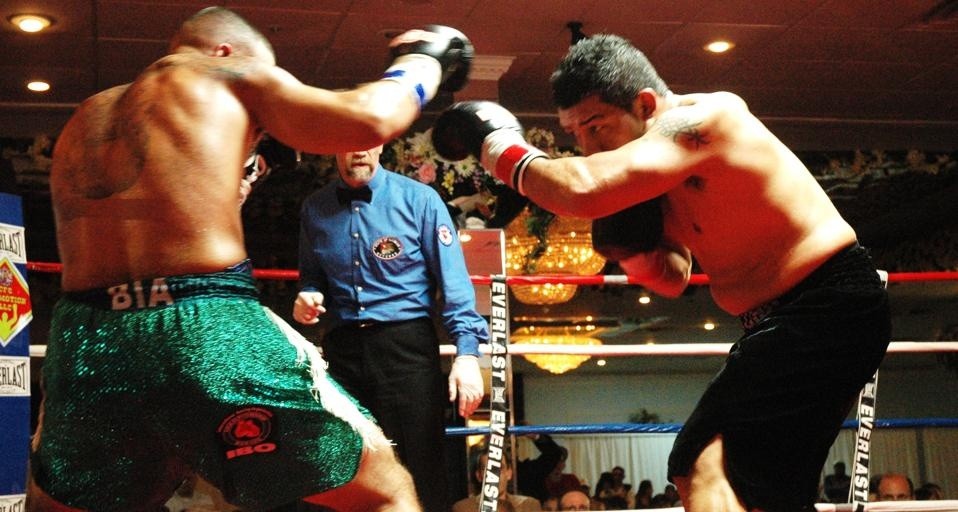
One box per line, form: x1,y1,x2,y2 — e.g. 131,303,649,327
375,21,480,112
587,200,670,287
430,97,551,201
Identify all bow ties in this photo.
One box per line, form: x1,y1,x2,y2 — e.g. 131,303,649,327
334,184,373,206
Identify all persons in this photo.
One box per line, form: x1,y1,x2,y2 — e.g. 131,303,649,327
289,140,494,512
452,418,942,511
433,32,899,511
23,3,476,512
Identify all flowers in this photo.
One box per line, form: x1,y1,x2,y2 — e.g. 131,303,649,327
386,123,573,206
